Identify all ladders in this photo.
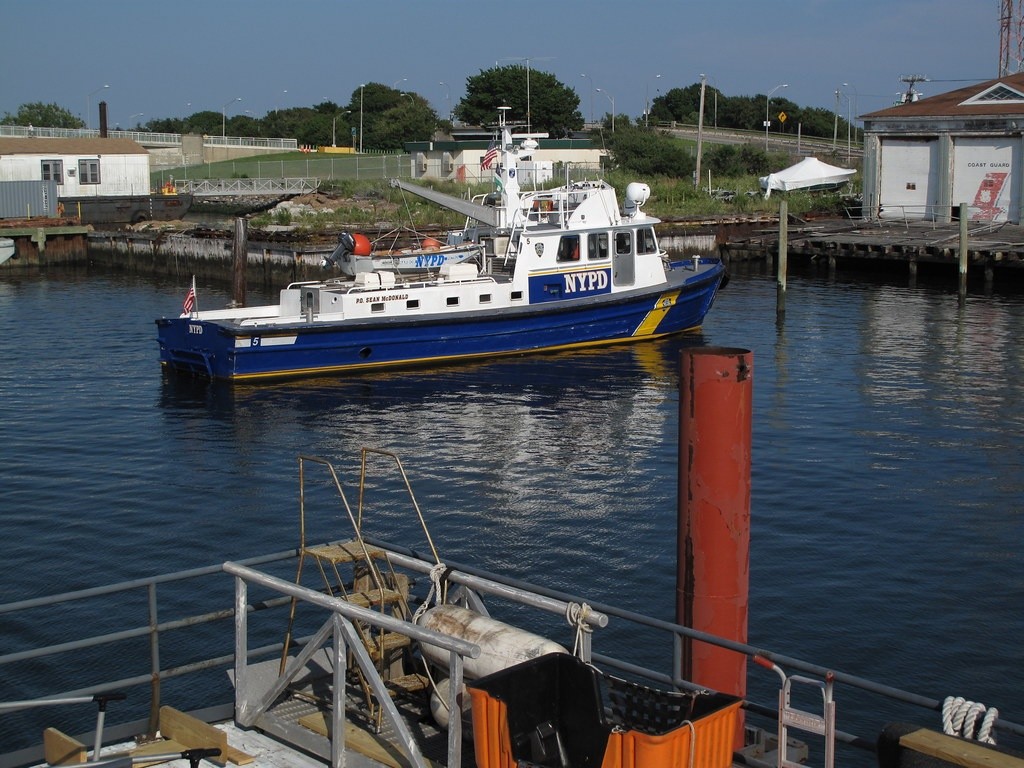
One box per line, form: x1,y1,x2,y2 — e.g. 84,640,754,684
279,446,450,735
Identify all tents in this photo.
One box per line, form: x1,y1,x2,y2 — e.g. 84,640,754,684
759,157,858,197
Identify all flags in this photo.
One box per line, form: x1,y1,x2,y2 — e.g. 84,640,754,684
183,279,196,314
480,137,498,171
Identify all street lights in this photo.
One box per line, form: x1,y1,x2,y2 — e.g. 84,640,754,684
178,103,190,121
360,85,365,153
245,110,260,132
766,84,788,153
843,82,858,144
222,97,241,139
400,93,415,106
88,84,110,138
645,75,661,130
580,73,594,123
275,90,288,115
835,89,851,166
129,113,144,132
439,82,451,115
700,73,717,130
596,88,614,131
393,79,407,89
333,111,351,146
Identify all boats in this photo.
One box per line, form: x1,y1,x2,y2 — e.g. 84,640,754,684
154,127,725,378
758,157,859,197
